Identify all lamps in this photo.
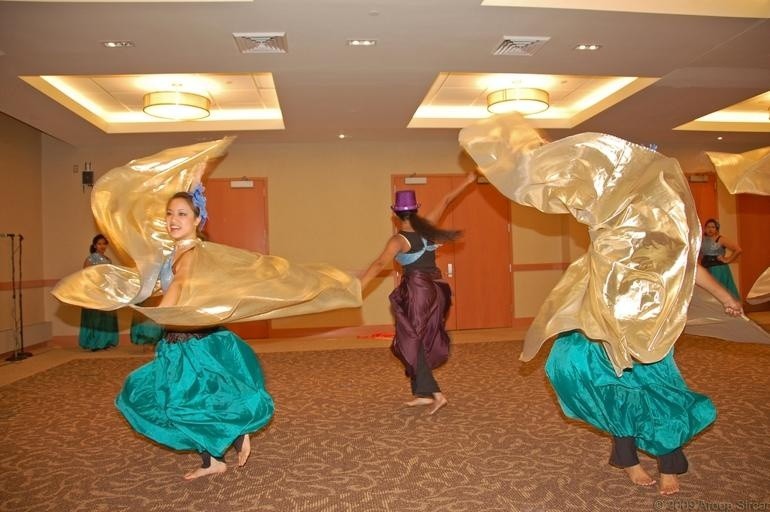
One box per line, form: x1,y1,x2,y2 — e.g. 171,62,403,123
485,88,554,119
141,92,215,124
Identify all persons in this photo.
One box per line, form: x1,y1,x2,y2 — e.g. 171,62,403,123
459,112,749,496
78,234,119,350
360,167,478,417
700,216,744,304
48,132,363,480
129,295,165,347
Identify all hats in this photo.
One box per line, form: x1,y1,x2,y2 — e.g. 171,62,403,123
392,190,419,212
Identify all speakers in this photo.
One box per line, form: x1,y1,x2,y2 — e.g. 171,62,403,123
82,171,94,185
